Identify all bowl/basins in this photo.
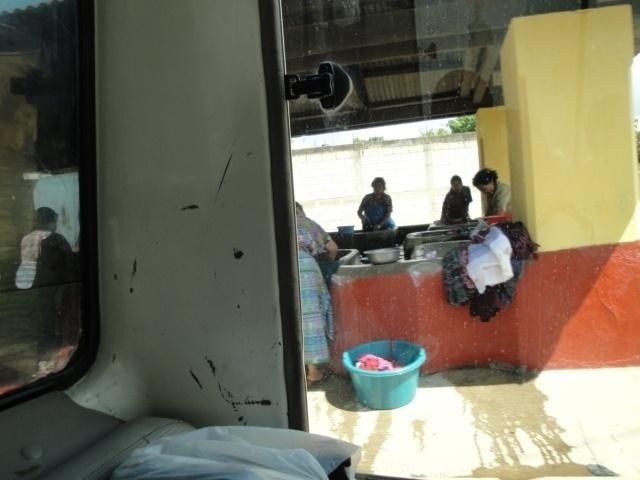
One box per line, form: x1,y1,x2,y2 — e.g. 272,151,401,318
485,214,512,226
338,226,355,233
363,248,401,265
343,338,428,412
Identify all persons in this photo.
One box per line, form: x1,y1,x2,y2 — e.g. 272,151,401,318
357,177,397,232
441,175,473,224
473,169,512,217
13,206,72,375
295,203,339,386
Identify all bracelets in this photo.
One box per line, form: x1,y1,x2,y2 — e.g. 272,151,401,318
378,224,381,229
